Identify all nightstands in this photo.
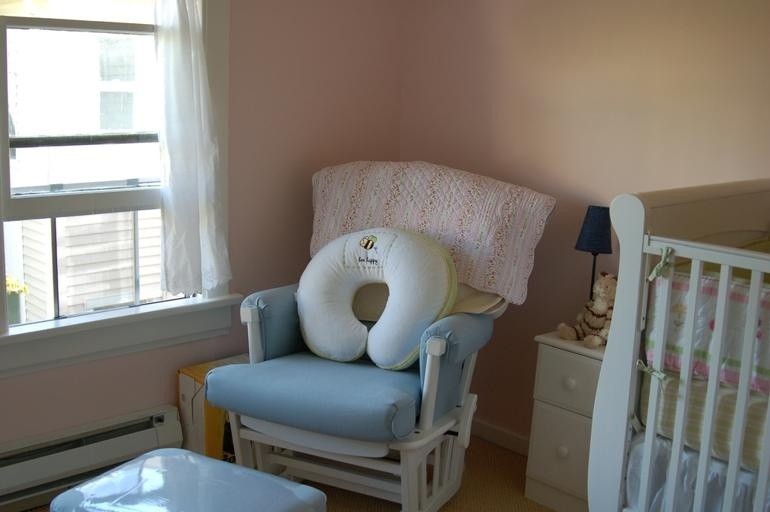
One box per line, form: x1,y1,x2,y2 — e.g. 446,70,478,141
524,320,606,511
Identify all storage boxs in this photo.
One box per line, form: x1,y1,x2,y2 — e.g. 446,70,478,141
175,352,250,462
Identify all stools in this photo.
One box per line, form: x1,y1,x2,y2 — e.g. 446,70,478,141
47,447,328,512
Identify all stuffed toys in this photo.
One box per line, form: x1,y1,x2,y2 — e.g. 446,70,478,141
557,272,617,348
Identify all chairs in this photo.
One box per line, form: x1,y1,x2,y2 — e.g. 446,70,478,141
203,157,558,511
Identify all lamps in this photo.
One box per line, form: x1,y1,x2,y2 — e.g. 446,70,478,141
570,204,611,302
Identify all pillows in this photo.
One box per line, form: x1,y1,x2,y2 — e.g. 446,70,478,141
295,223,459,369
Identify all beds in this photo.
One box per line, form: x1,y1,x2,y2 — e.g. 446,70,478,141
585,178,769,512
